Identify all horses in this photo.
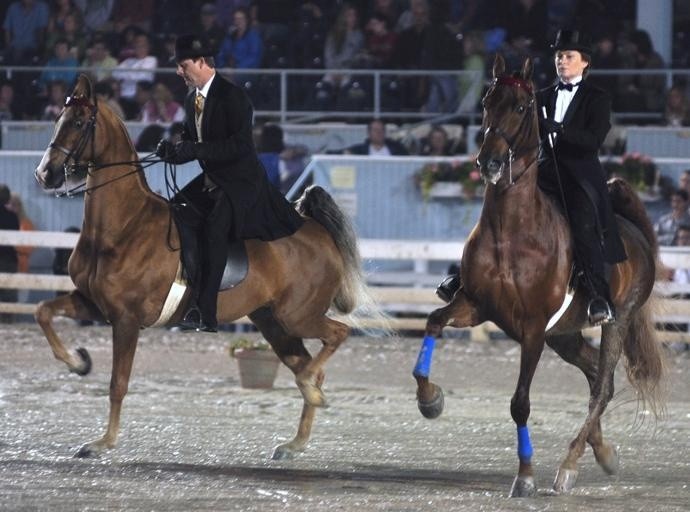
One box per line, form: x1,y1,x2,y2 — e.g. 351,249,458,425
407,49,665,499
29,69,397,463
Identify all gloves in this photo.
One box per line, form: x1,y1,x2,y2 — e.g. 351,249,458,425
175,140,216,161
542,118,566,134
155,140,176,162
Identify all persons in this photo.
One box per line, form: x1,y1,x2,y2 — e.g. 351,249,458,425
152,34,303,334
3,191,36,272
1,0,689,127
133,123,310,160
602,135,689,250
0,184,20,331
326,118,465,157
433,27,626,327
52,227,83,297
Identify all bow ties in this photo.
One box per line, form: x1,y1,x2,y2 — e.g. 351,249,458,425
559,81,581,91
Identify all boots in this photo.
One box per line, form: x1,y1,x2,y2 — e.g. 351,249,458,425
438,275,461,302
178,288,219,332
585,273,616,325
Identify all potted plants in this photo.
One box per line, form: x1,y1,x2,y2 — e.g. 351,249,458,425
230,337,280,390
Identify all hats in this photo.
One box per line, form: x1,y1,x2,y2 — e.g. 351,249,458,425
550,29,592,54
174,35,213,58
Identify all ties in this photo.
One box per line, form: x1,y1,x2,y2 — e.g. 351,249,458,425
194,96,202,114
